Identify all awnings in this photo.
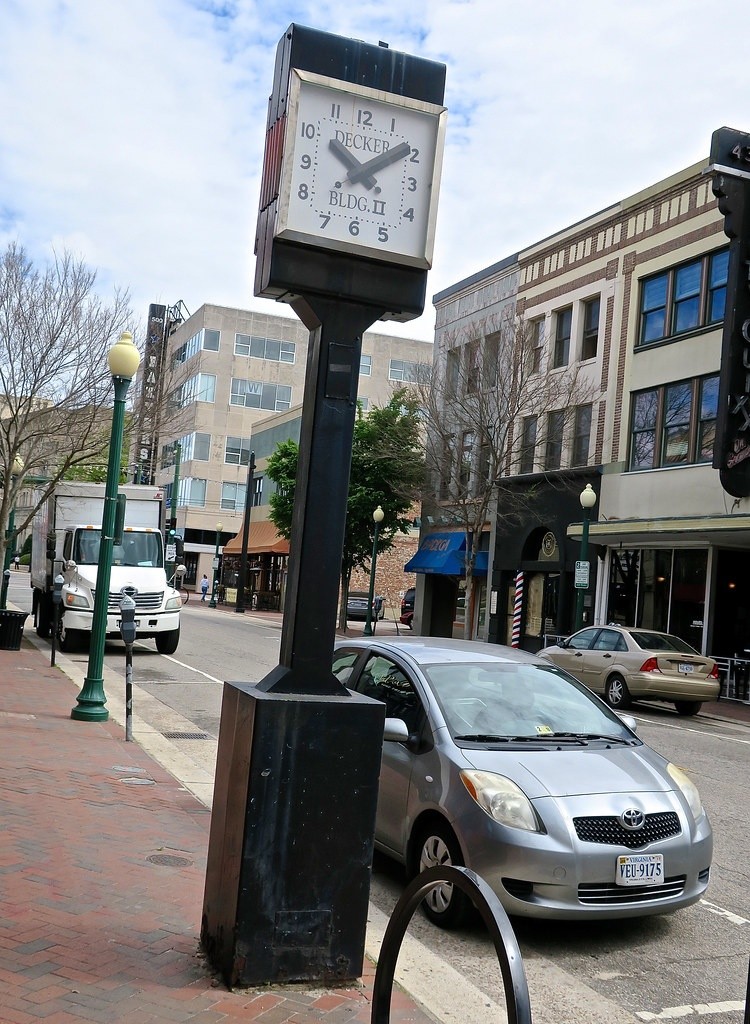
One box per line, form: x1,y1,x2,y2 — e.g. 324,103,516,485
222,521,290,553
403,532,489,578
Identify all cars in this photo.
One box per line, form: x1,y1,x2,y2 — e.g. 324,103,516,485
534,622,720,717
348,590,387,622
331,636,713,932
400,611,415,630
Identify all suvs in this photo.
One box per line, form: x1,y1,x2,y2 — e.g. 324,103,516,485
400,586,415,615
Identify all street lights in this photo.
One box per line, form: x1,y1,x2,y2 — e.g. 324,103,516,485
209,521,224,608
362,505,385,637
574,483,597,632
71,327,141,722
0,452,25,609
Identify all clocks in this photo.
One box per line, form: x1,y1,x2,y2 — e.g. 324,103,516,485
271,67,447,271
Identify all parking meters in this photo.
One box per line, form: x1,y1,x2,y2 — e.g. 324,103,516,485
0,568,11,609
118,595,137,741
51,574,65,667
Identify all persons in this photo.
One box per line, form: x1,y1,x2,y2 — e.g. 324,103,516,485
15,555,20,570
200,574,209,602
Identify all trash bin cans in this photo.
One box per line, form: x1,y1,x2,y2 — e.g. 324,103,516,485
0,608,29,652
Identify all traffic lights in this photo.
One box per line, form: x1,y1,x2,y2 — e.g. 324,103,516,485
170,517,178,536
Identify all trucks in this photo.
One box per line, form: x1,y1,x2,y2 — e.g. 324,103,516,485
30,480,185,653
